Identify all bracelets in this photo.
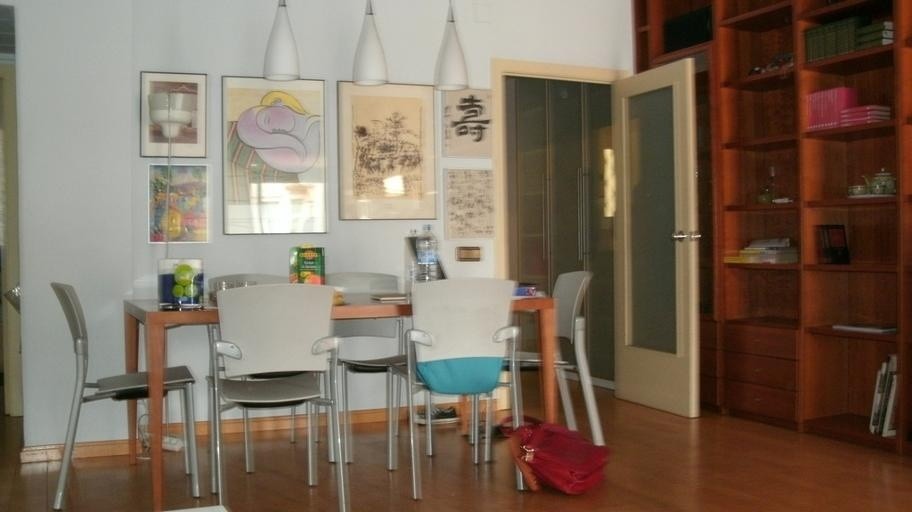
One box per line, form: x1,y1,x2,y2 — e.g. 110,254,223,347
513,284,535,295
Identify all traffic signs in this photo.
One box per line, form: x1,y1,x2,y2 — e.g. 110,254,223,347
143,433,183,452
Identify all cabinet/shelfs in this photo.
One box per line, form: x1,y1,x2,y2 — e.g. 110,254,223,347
757,187,773,205
767,166,776,203
417,225,438,282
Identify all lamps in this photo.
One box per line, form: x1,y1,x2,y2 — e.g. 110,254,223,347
387,277,529,500
326,272,432,464
50,281,200,511
209,273,327,495
503,270,604,446
207,283,349,512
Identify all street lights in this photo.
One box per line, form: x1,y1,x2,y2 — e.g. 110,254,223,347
498,415,614,492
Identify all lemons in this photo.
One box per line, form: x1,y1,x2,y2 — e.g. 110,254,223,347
861,168,896,194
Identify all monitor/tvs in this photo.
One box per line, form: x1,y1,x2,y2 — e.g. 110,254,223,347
723,237,799,264
804,17,893,62
832,324,898,334
370,292,408,304
814,224,851,264
868,352,898,438
807,86,890,130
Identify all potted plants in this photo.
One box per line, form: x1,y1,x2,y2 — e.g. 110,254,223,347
221,76,327,234
139,71,207,158
337,80,438,220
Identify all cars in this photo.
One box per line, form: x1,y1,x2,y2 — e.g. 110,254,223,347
847,186,865,195
209,280,234,301
236,281,256,288
871,185,884,193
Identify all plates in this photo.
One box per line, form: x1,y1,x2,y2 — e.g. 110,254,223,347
632,0,910,451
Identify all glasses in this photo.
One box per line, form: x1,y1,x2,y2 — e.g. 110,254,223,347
436,2,469,91
264,0,301,81
352,1,390,85
